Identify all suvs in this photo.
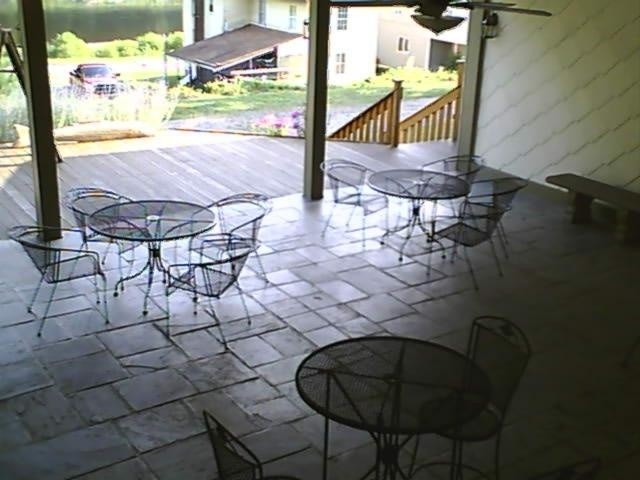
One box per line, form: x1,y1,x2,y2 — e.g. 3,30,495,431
69,63,124,100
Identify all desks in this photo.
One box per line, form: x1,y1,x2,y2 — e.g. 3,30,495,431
87,200,221,318
294,334,495,480
367,168,472,266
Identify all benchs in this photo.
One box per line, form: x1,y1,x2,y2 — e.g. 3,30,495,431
545,173,640,242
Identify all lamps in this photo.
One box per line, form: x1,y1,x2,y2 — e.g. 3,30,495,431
481,13,498,40
410,15,465,36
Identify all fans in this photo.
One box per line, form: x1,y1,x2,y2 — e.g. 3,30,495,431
323,0,553,20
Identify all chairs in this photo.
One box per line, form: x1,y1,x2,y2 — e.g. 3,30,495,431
412,150,489,229
315,155,395,250
61,184,154,300
185,191,274,289
405,315,532,480
164,235,257,359
529,455,604,479
199,404,316,480
424,199,510,293
4,220,115,337
457,176,530,261
